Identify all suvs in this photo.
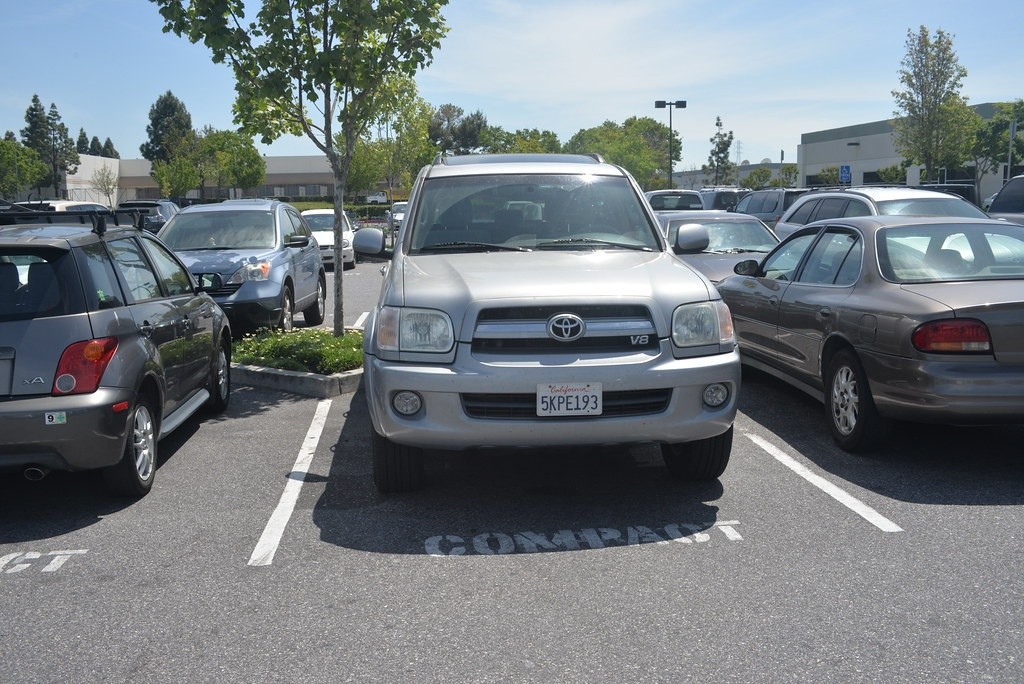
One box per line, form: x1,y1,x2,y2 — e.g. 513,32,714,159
116,199,180,234
156,199,326,330
702,184,992,244
0,215,231,497
363,149,740,495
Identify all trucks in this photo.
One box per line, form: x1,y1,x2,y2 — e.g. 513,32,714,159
365,188,411,205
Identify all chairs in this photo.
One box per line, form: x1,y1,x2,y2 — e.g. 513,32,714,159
932,247,971,274
424,196,493,244
822,250,861,284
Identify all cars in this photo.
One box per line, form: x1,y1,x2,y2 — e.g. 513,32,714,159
301,209,357,270
717,214,1024,455
645,191,706,210
0,198,112,213
654,209,781,289
505,201,533,213
987,174,1024,217
385,202,408,230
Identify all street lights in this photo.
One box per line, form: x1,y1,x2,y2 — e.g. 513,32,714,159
654,98,687,190
